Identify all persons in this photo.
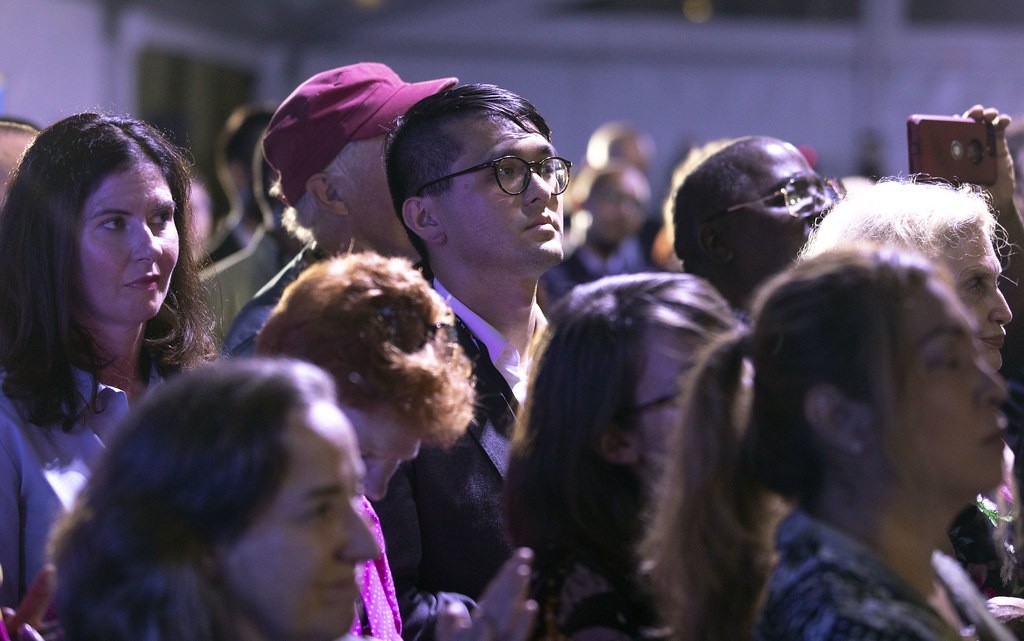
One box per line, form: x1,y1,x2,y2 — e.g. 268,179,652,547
636,243,1016,641
0,61,1024,641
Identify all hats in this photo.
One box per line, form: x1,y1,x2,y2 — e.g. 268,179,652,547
259,62,460,207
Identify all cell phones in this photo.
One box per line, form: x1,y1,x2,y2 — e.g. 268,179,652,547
907,115,997,186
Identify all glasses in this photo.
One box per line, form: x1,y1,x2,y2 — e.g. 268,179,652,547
341,295,478,376
415,155,573,196
691,171,847,244
